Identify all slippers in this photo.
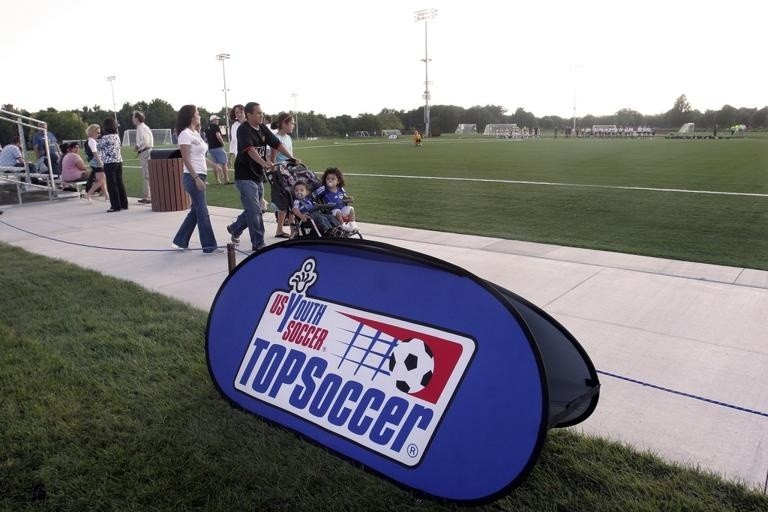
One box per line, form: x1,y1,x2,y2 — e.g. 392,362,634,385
274,231,290,239
202,248,224,255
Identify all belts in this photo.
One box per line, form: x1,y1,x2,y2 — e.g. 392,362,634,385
137,148,149,154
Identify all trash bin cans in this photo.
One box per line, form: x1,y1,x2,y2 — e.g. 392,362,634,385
147,149,192,213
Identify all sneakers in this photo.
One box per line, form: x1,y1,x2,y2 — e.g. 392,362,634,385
107,207,120,212
137,198,147,202
141,199,151,203
227,223,241,245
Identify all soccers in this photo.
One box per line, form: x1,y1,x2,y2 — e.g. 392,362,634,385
388,337,435,395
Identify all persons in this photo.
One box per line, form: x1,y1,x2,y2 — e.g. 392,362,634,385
96,118,128,212
171,105,224,254
132,110,154,203
33,121,61,160
226,104,268,211
58,142,87,183
206,115,235,184
291,181,349,238
226,103,301,252
269,113,296,238
82,124,111,204
0,136,38,185
314,168,357,231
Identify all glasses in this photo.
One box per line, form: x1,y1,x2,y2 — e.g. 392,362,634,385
256,112,265,115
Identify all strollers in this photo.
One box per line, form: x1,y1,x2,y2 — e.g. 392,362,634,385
262,158,363,239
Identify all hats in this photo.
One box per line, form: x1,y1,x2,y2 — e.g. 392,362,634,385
209,114,221,121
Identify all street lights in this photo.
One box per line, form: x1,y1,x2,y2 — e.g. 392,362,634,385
413,7,438,137
106,76,118,132
215,53,230,142
